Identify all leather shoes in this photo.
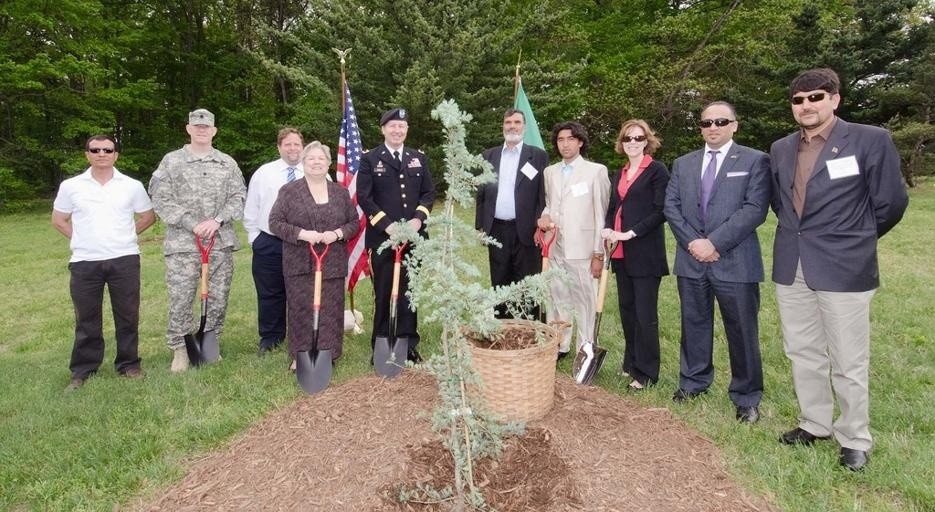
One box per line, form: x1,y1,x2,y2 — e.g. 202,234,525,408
119,367,145,378
288,360,296,374
736,404,759,424
626,378,645,392
674,388,698,404
559,351,568,359
840,446,868,470
259,339,277,354
63,378,84,392
779,427,831,446
407,348,422,364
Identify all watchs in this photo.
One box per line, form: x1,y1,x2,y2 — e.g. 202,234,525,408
213,214,224,228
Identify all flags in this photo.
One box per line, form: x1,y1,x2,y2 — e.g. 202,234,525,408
339,75,377,291
515,78,547,151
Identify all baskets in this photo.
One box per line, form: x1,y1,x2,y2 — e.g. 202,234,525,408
460,319,572,425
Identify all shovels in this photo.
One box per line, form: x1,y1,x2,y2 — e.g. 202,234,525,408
573,239,619,385
373,240,408,379
296,244,332,394
184,236,219,367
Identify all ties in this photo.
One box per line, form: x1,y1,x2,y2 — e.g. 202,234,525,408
393,151,401,166
287,167,296,183
702,151,719,223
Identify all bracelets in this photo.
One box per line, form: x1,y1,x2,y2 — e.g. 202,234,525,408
629,231,635,240
332,229,340,241
594,255,605,261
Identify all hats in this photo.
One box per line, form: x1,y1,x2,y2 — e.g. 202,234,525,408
188,109,216,127
380,108,409,126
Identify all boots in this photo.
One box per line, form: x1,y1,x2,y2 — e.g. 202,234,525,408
171,346,190,373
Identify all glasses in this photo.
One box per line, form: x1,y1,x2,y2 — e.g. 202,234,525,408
699,118,734,128
622,136,647,142
791,93,832,105
89,147,113,154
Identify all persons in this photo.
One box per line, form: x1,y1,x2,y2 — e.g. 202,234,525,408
356,108,437,365
475,109,551,324
598,117,673,393
536,120,612,362
664,100,771,424
768,67,911,473
149,108,243,375
243,128,337,359
51,135,155,393
267,140,361,374
